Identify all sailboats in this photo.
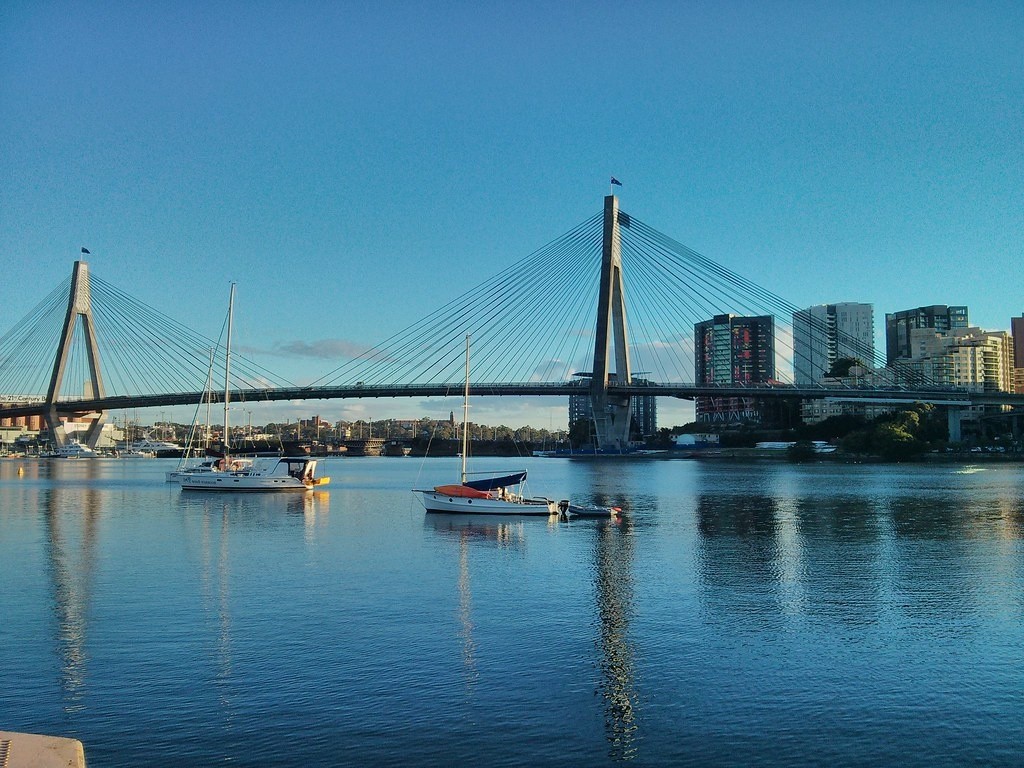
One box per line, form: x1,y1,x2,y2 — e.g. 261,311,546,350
166,347,261,484
177,280,330,491
411,332,559,515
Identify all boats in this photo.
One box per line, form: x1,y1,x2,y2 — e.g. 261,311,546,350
127,434,189,457
57,439,99,458
568,504,622,516
40,450,61,458
